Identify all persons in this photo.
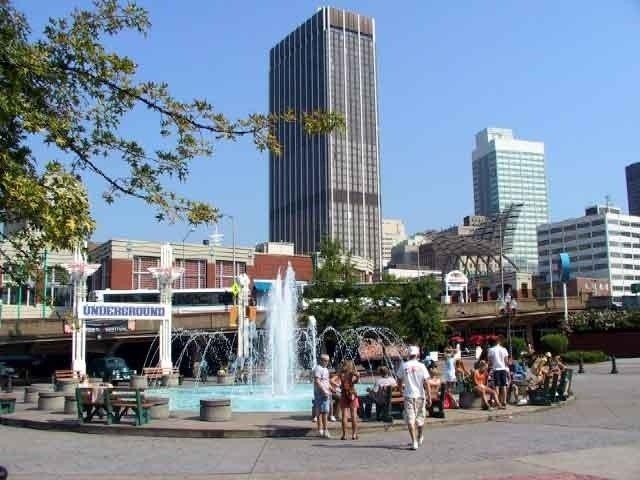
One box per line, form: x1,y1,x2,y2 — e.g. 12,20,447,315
311,335,570,450
78,376,113,418
193,359,207,381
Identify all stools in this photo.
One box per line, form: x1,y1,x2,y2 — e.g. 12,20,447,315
23,382,170,420
199,398,232,422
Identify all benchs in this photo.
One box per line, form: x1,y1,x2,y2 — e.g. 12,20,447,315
142,365,184,388
52,367,87,391
376,382,447,424
75,386,155,427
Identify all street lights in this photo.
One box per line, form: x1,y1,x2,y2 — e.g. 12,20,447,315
183,228,195,289
499,293,517,360
224,215,235,288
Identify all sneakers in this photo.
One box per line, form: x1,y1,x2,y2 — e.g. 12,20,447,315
410,441,418,449
418,435,424,446
341,432,348,440
312,416,317,422
352,432,358,440
329,415,336,421
319,430,330,438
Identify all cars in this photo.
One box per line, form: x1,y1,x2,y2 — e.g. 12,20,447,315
89,357,137,385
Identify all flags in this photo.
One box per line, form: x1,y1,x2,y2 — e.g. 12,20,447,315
232,283,239,295
